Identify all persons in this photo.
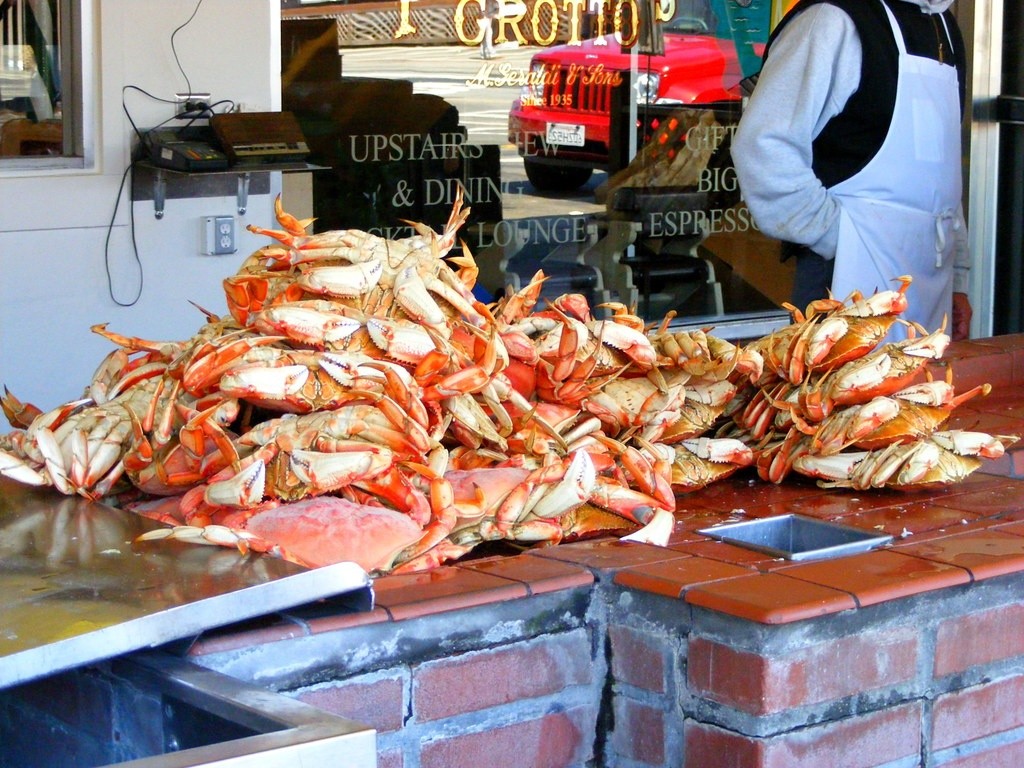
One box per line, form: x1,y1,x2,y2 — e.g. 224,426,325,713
729,0,973,352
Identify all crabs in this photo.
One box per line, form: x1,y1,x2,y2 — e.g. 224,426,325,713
0,183,1022,577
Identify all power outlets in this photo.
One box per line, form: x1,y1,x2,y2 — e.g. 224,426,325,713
174,93,211,120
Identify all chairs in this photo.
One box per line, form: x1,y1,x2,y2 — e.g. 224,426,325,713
498,224,612,320
611,185,725,316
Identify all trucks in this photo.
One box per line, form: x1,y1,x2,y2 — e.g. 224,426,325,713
506,1,796,196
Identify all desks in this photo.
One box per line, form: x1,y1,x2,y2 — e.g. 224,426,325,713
465,192,608,236
